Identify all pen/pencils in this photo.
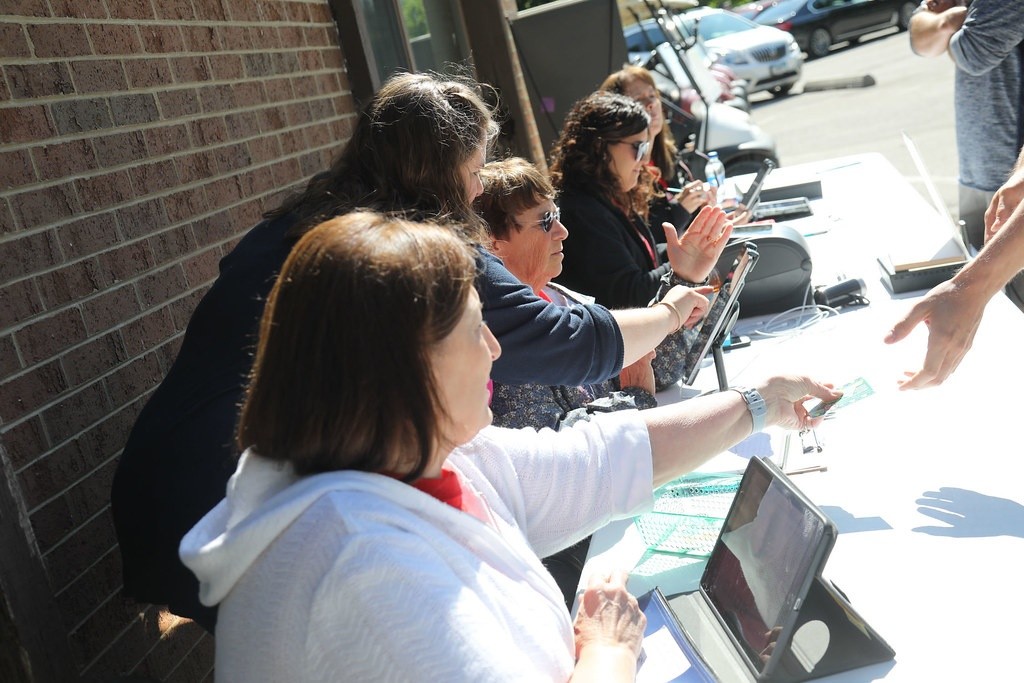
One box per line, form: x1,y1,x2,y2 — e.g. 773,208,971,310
803,230,828,237
666,186,684,194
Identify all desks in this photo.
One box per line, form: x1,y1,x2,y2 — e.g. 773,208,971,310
572,146,1023,683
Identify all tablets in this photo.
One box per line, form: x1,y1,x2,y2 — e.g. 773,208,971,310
738,157,775,211
698,454,833,682
681,241,759,387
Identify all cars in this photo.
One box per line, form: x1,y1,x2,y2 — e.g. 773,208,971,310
733,0,923,58
625,5,804,102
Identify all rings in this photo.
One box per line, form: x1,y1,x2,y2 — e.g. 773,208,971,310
707,236,718,244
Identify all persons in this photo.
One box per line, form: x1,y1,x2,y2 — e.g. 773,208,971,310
468,158,734,433
883,0,1024,390
180,207,843,683
549,88,748,311
599,66,716,246
110,65,717,635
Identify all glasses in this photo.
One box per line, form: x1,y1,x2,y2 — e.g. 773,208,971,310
512,207,561,233
617,140,650,162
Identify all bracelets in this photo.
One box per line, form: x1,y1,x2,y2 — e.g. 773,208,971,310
658,302,680,333
731,385,768,433
